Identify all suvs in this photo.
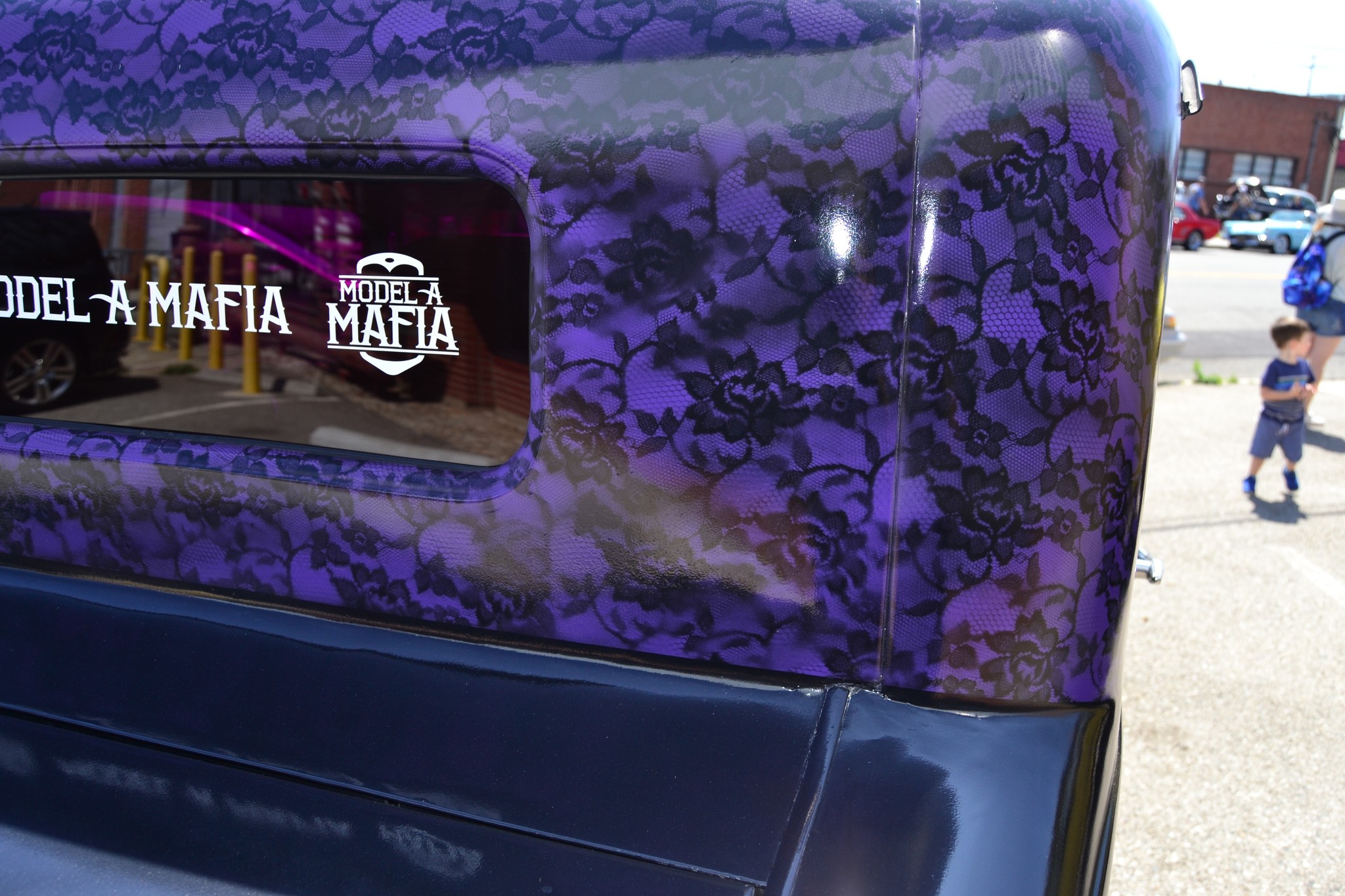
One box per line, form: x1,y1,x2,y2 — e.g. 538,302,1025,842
1,203,134,418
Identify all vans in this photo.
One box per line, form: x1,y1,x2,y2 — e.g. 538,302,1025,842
1,1,1203,896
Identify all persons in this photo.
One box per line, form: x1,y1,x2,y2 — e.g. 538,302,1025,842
1296,188,1345,424
1223,183,1263,222
1288,195,1304,210
1242,315,1316,494
1187,175,1214,218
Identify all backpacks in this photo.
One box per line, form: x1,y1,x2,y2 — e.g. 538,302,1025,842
1281,231,1345,311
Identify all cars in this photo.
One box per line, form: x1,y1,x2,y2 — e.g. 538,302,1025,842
1169,200,1220,252
1222,209,1317,254
1261,185,1317,215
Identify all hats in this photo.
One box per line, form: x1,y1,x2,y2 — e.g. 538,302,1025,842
1314,187,1345,226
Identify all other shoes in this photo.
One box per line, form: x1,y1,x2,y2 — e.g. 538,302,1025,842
1282,467,1298,490
1301,412,1325,427
1243,475,1256,494
